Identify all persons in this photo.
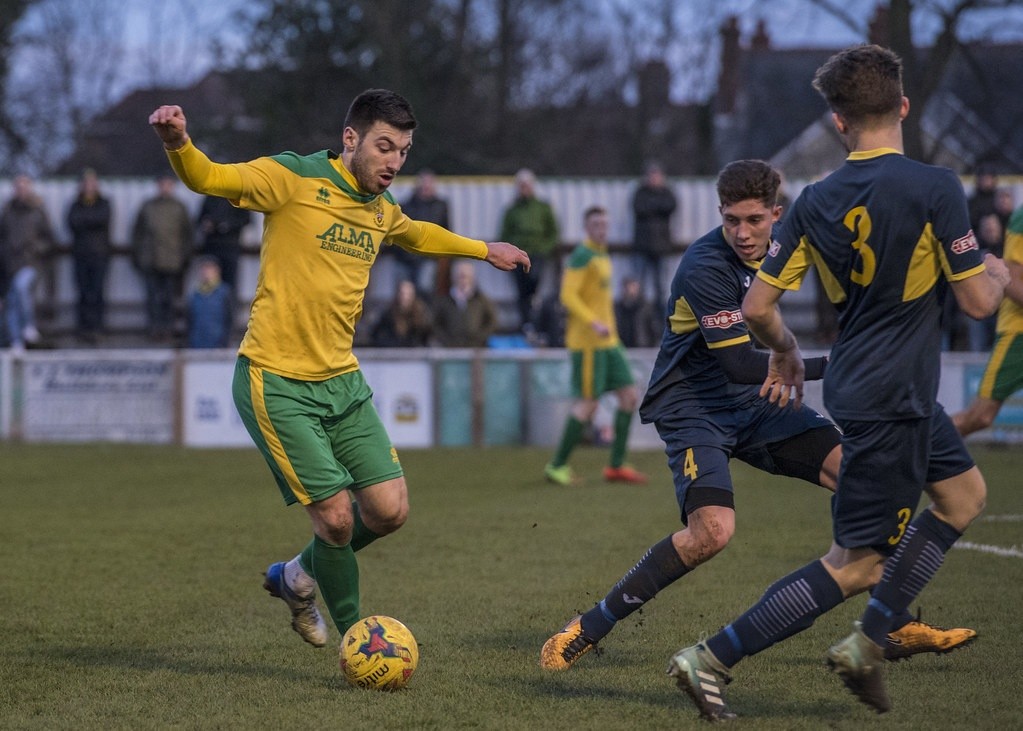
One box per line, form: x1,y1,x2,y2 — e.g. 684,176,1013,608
197,196,250,288
372,276,432,347
432,256,497,347
936,164,1014,350
631,157,675,254
148,88,532,646
131,171,196,349
392,170,451,296
500,167,558,325
0,171,58,352
183,254,233,348
66,165,111,340
540,155,1023,673
614,275,661,350
669,44,1010,725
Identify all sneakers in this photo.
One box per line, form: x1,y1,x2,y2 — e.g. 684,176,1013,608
883,606,977,662
540,614,599,674
825,629,889,715
263,563,327,647
665,641,737,722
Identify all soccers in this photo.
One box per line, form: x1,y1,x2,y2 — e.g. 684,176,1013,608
339,615,419,691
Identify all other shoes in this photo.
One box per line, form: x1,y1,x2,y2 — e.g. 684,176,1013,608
543,462,572,487
603,465,648,484
